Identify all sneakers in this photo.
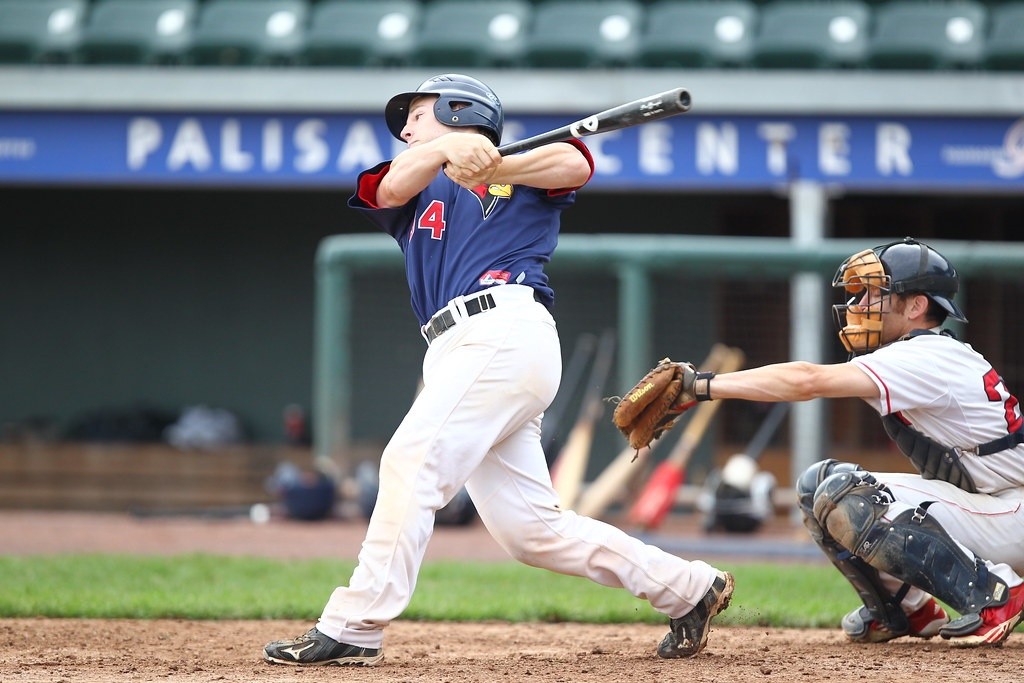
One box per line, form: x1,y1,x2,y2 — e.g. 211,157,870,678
657,571,733,660
949,582,1024,648
263,626,385,667
847,598,951,643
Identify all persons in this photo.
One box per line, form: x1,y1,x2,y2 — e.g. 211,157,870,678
668,239,1023,648
262,74,735,668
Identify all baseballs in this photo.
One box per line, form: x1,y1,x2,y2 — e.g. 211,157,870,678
251,501,273,523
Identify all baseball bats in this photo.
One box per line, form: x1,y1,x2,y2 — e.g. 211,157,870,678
441,85,693,178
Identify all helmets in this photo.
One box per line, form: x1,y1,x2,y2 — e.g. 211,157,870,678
872,236,968,323
385,74,504,146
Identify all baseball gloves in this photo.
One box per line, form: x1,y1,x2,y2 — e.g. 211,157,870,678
605,356,716,464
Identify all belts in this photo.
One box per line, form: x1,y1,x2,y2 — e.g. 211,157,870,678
424,290,542,343
